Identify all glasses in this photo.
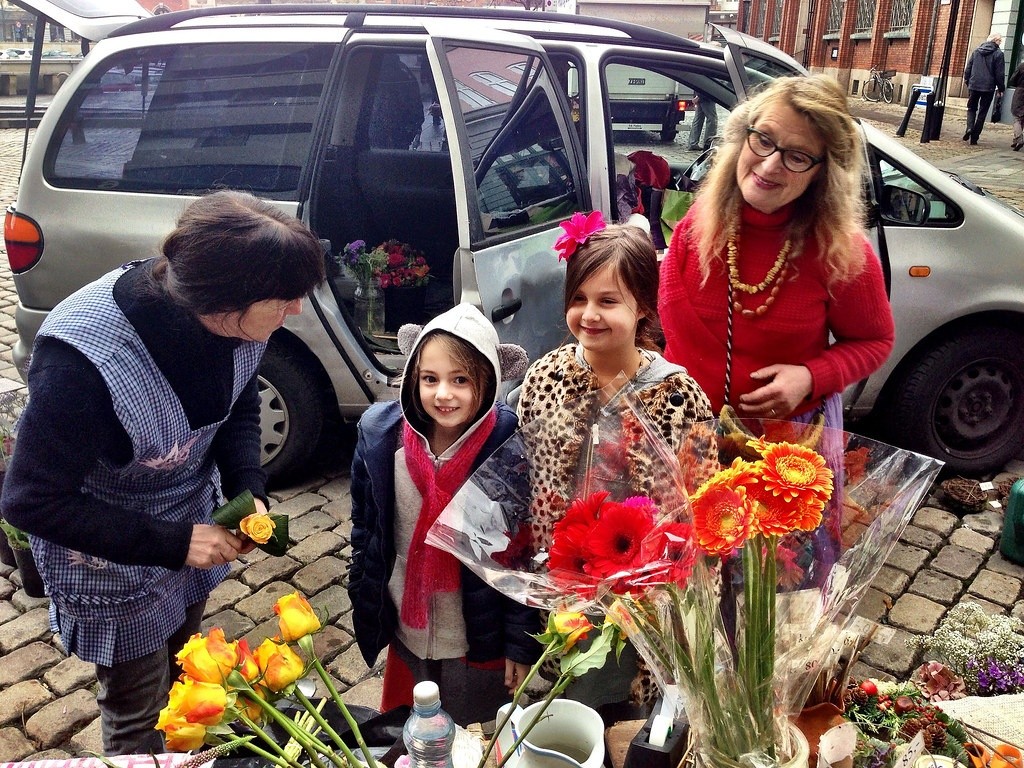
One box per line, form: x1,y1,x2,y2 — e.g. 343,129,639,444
746,118,828,174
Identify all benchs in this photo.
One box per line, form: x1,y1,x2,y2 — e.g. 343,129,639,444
321,142,454,333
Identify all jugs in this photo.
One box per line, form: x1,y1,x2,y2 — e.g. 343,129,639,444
495,699,608,768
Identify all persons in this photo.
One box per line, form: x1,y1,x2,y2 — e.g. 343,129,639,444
0,190,327,757
348,303,544,741
963,33,1005,145
1008,62,1024,151
657,74,895,672
516,210,721,768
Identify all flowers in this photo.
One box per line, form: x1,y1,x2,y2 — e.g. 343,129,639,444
76,587,379,768
477,436,1024,768
211,490,290,558
0,390,31,549
338,239,438,332
553,212,606,262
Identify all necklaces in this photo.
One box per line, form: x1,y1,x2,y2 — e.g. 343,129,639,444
727,196,792,319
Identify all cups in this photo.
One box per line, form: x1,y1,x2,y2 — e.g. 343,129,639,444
819,721,938,768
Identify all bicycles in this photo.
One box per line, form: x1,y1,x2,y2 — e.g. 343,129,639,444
862,63,897,104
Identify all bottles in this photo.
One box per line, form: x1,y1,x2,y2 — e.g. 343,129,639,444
402,681,456,768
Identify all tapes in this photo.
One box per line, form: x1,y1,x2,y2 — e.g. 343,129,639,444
647,685,680,747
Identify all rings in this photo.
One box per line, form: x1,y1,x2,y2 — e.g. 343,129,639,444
772,409,776,415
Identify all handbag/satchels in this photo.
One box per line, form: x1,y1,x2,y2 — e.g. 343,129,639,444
991,89,1002,123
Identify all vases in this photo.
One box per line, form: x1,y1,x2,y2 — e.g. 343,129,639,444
8,541,50,598
696,719,810,768
0,527,17,568
816,721,937,768
351,282,385,335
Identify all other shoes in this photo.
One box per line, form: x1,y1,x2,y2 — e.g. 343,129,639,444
970,140,977,144
962,128,972,141
1011,142,1023,151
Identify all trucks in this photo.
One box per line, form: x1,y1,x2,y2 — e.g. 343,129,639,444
555,0,710,141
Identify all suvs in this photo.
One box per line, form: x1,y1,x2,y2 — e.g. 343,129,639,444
2,0,1024,481
